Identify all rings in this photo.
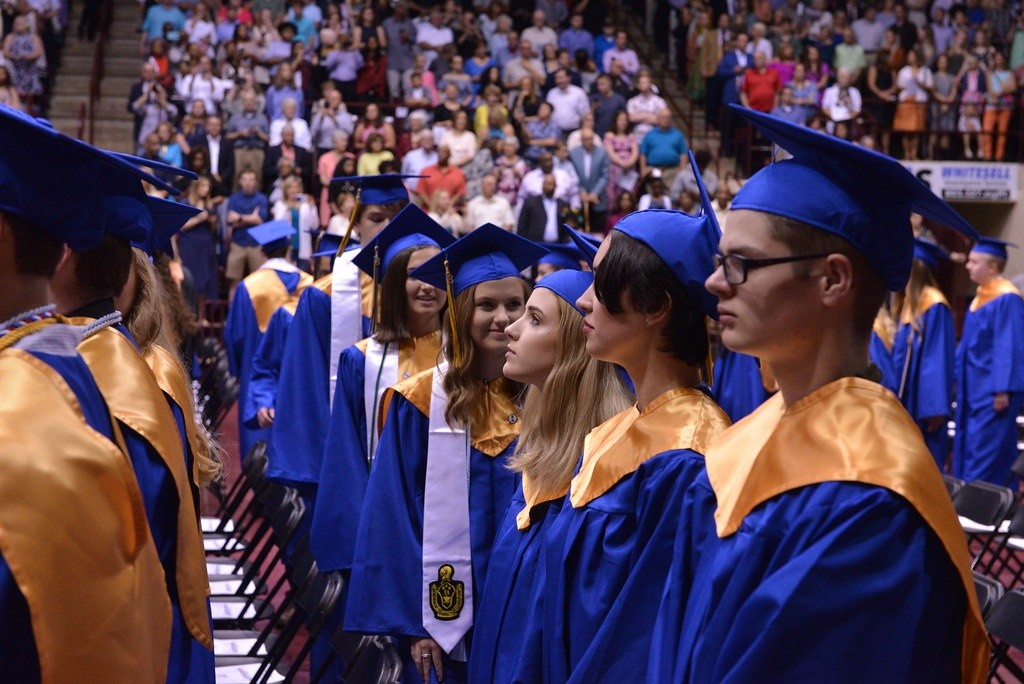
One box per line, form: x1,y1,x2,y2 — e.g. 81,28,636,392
423,654,431,657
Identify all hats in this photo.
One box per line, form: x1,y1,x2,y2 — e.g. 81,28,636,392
614,149,729,321
330,174,432,258
535,223,608,318
351,201,459,335
311,235,360,258
409,222,549,370
535,242,582,271
246,218,297,249
966,234,1017,258
726,101,978,294
0,103,203,260
912,235,951,272
579,233,602,248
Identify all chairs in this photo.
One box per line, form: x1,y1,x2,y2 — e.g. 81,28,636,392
190,336,404,684
938,448,1024,684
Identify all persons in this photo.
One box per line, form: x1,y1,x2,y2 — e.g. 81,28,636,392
671,0,1024,158
308,204,460,571
644,104,992,684
264,174,434,482
0,0,61,107
868,240,955,470
342,223,550,684
49,152,228,684
129,0,732,276
540,150,733,684
465,223,639,684
0,110,181,684
953,237,1024,486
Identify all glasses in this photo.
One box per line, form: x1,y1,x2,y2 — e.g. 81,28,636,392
712,248,833,286
